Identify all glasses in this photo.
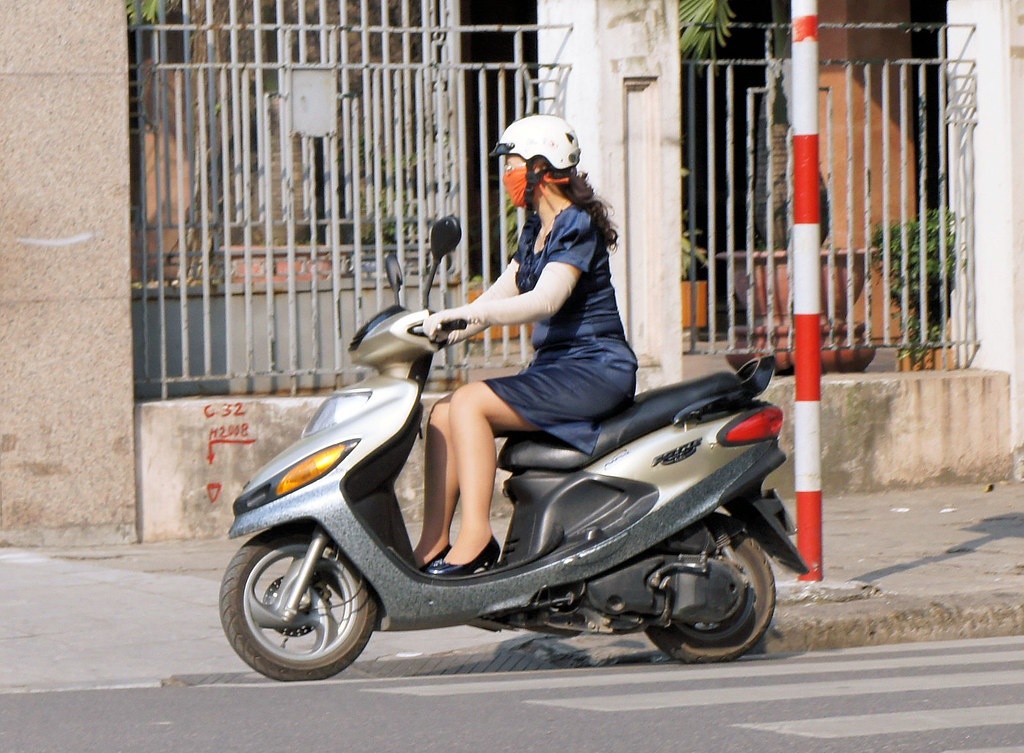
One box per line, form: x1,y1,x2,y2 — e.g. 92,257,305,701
502,163,526,174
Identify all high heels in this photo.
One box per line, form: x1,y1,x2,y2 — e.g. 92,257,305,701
418,545,453,574
426,534,501,576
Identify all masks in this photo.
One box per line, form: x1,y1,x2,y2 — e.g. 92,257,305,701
502,169,570,206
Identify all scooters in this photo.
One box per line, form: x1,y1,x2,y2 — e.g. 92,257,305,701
217,216,810,685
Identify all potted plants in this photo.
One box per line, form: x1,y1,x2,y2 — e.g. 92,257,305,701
871,204,966,371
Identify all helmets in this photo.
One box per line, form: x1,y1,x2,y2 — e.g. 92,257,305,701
488,115,582,170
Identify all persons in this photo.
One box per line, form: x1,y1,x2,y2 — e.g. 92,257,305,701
411,114,638,577
